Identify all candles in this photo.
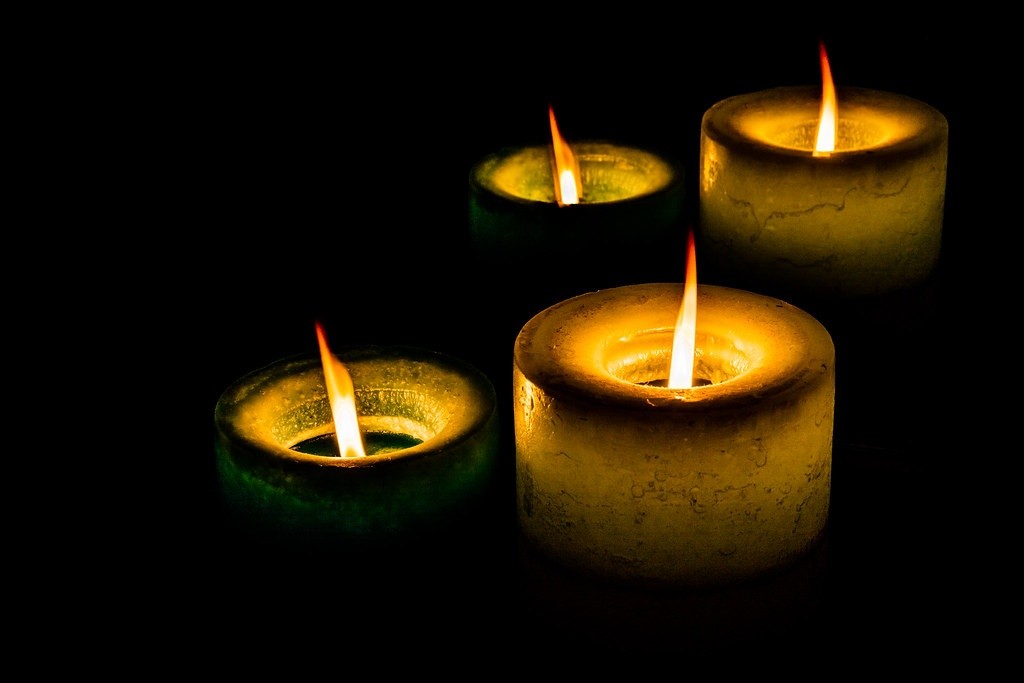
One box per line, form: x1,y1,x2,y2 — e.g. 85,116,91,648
510,228,838,581
213,319,500,532
465,100,687,242
698,40,950,289
